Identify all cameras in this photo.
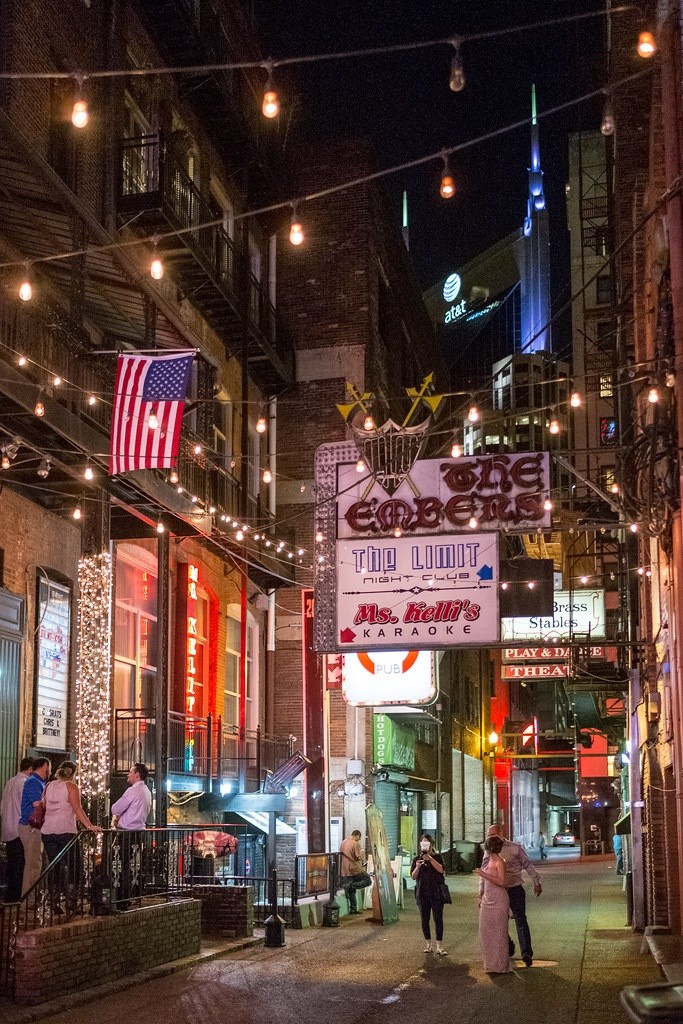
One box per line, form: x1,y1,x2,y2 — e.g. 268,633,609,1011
420,859,428,868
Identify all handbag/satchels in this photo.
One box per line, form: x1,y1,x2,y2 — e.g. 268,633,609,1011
440,884,452,904
28,782,51,831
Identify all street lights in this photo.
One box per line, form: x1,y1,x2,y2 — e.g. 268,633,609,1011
488,729,500,829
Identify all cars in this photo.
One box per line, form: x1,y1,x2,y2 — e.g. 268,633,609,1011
553,831,577,848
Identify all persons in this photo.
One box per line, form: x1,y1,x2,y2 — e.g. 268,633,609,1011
340,830,372,915
111,763,152,911
478,825,542,968
473,835,514,973
41,761,102,915
1,757,52,910
410,834,447,955
539,831,548,860
613,834,623,875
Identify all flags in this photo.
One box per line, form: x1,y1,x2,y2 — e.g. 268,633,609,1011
108,353,196,475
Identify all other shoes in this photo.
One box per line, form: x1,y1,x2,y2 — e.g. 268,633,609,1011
350,909,363,914
424,947,433,953
343,884,350,898
436,949,447,955
509,942,515,957
522,954,532,967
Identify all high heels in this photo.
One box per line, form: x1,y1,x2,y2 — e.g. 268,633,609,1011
48,904,64,914
65,902,86,915
